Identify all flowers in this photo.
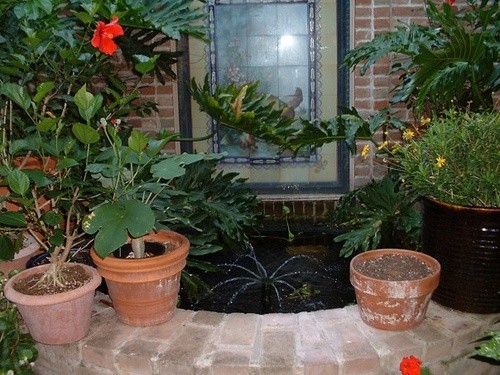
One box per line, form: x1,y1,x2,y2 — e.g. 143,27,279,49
360,99,500,210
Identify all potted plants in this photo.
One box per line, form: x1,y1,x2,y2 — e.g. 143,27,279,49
81,145,229,327
0,52,182,345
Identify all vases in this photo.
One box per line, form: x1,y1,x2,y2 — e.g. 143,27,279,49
419,195,500,315
